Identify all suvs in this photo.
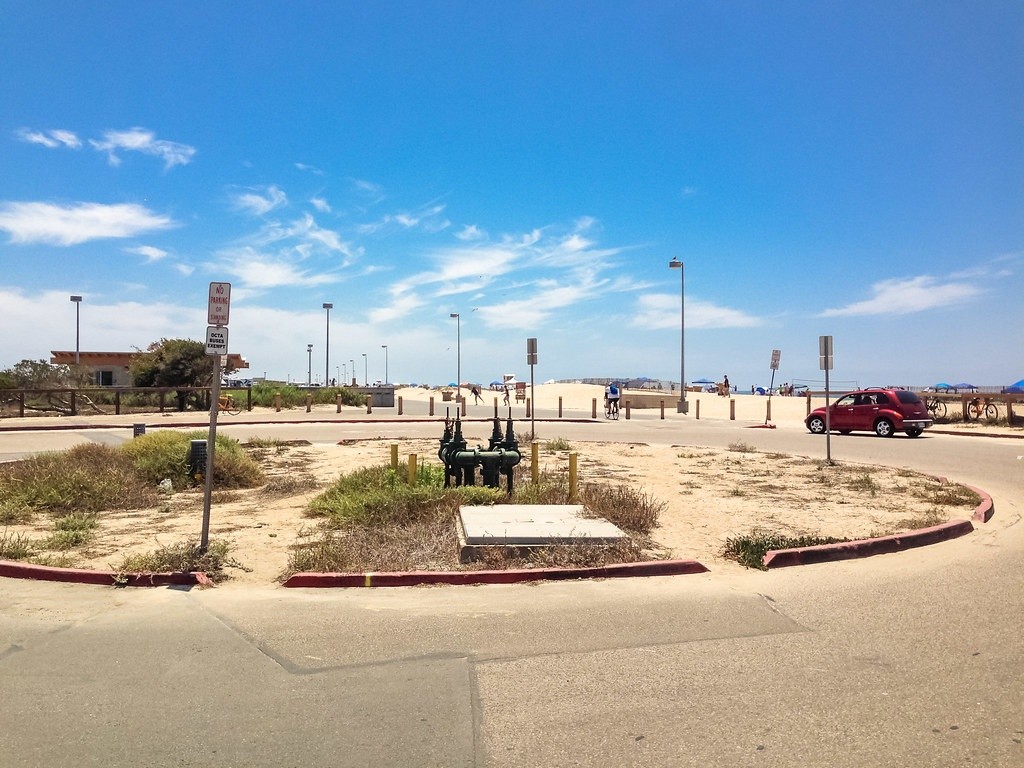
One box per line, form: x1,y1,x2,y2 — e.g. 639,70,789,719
803,386,933,439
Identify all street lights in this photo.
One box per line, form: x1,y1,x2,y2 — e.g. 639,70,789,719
449,313,462,402
323,302,333,388
381,345,388,386
336,363,347,386
362,353,368,385
306,343,313,386
669,254,690,414
70,295,83,364
350,359,355,378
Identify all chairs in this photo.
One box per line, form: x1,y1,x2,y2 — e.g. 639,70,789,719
855,396,864,403
863,397,871,403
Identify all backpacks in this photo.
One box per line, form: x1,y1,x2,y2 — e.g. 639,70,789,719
610,386,618,394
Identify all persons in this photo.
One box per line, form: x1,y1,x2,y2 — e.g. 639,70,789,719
470,387,485,406
1000,386,1007,394
605,382,620,414
722,374,730,398
777,382,793,396
751,385,754,395
502,385,510,406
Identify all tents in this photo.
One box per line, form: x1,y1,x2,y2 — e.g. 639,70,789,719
490,380,504,389
929,382,953,393
954,383,978,394
505,378,518,388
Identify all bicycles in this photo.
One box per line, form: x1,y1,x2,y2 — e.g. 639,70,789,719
208,390,242,416
920,396,948,421
605,401,620,421
967,397,999,421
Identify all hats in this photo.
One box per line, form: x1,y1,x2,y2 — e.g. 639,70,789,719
609,382,612,385
